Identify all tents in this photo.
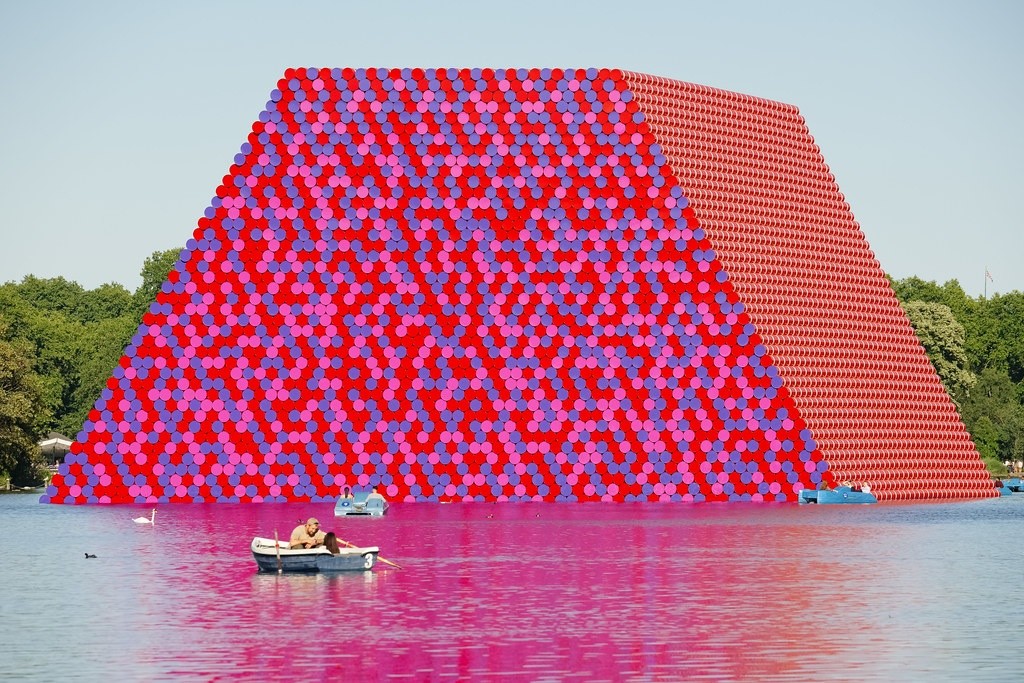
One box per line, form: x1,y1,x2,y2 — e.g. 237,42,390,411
36,438,73,463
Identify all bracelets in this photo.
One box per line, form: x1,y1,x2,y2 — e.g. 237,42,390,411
314,539,317,543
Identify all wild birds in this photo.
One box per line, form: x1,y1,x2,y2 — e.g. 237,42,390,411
132,509,158,523
487,514,493,518
85,553,97,559
536,512,541,517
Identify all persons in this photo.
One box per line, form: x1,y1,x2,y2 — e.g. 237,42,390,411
1004,459,1023,473
1019,476,1024,484
340,487,354,499
48,455,65,465
290,518,343,554
994,477,1004,488
366,485,388,507
820,482,838,493
858,481,871,493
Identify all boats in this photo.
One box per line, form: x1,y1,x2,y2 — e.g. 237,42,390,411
996,485,1013,496
250,536,380,573
1003,477,1024,492
334,490,384,517
798,487,878,506
36,431,75,458
